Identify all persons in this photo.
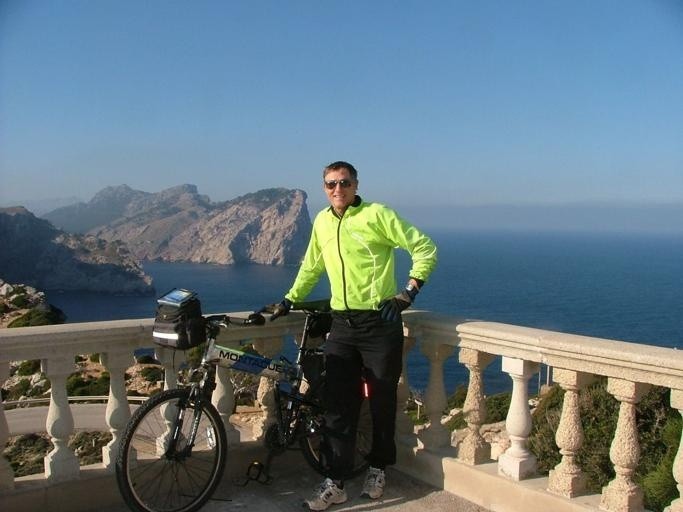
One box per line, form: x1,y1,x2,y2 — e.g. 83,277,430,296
254,160,438,512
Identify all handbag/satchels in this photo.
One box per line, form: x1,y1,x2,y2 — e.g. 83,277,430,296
151,288,207,350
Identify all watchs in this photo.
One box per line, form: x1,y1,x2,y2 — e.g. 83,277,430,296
405,284,418,295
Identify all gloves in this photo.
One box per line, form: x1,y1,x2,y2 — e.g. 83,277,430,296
378,288,413,321
255,300,291,321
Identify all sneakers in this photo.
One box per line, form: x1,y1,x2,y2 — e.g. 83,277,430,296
359,466,386,499
302,477,348,511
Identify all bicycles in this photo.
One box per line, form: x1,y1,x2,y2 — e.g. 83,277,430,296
113,287,397,511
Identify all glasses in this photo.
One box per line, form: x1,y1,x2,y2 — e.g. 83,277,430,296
324,179,351,189
